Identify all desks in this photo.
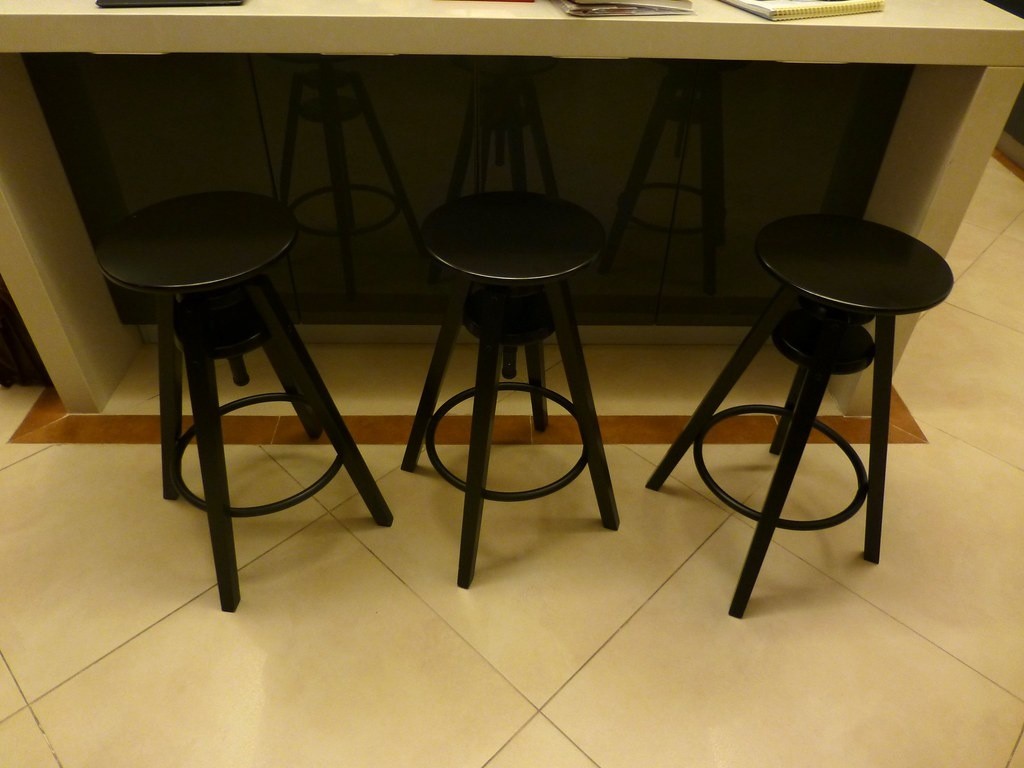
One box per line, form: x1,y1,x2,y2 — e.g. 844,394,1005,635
0,0,1024,417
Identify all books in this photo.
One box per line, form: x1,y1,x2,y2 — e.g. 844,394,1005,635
719,0,887,22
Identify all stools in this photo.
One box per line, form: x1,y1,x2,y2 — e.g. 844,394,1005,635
402,192,620,589
645,212,955,620
610,59,727,286
444,56,558,200
276,53,416,281
96,192,396,613
